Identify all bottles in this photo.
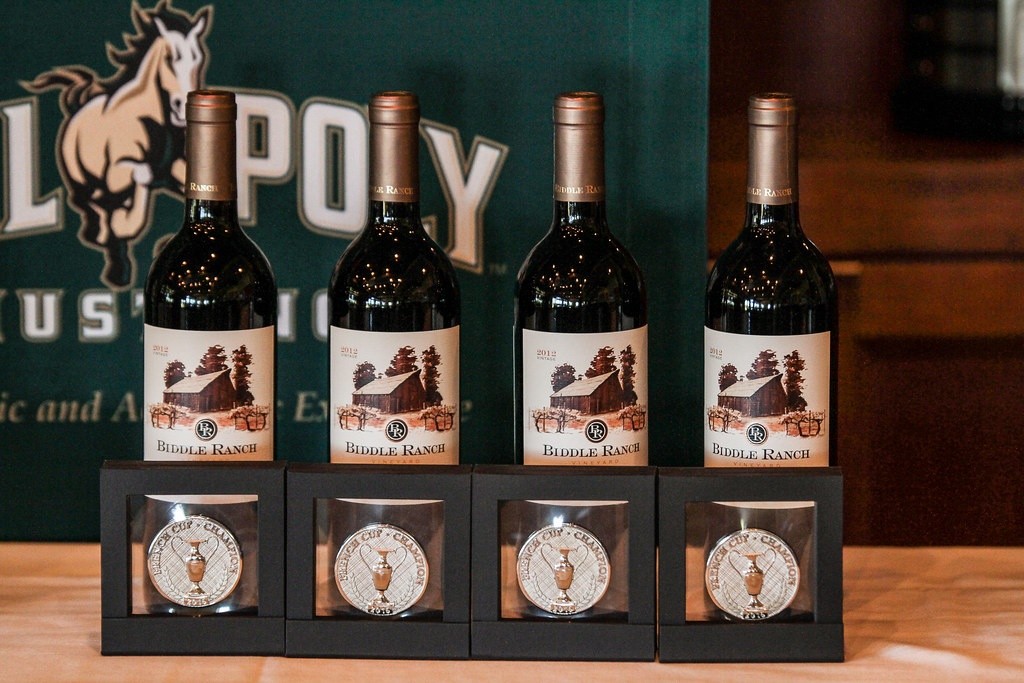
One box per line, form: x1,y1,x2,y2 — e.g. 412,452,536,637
328,90,463,619
142,90,277,615
702,91,838,620
512,86,650,618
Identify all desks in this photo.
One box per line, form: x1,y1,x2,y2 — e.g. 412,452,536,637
0,542,1023,683
